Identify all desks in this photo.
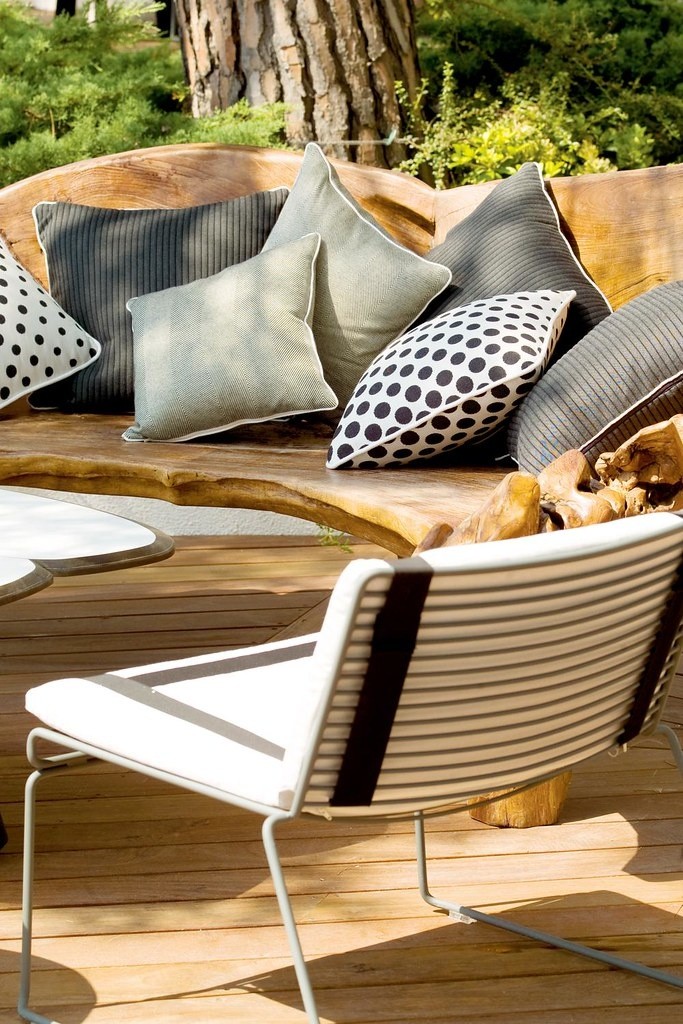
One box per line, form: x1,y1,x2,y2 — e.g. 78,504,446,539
0,490,177,606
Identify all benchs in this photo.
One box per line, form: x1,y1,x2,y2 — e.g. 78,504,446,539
0,143,683,828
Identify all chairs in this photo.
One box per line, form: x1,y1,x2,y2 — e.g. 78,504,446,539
18,510,682,1024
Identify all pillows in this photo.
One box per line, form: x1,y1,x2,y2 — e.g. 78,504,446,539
325,289,577,469
262,141,453,426
418,161,616,459
0,227,101,411
122,232,339,445
506,279,683,486
27,186,290,409
